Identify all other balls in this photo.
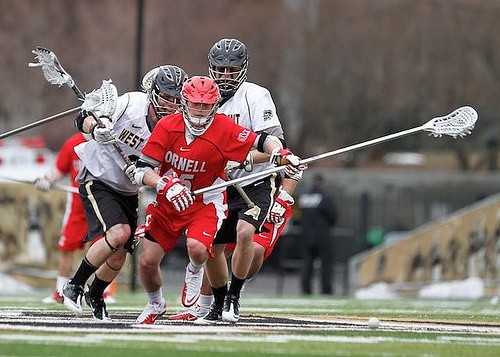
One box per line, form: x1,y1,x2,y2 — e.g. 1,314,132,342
369,317,380,329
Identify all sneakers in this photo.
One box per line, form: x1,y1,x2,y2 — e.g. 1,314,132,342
168,304,210,321
194,294,241,325
181,263,205,309
83,286,111,321
57,279,85,316
136,299,166,324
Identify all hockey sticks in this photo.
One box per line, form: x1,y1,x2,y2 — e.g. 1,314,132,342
141,67,254,210
0,171,157,208
0,77,119,140
192,104,478,196
27,44,133,167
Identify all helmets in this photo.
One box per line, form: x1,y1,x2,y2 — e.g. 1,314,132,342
208,36,249,97
149,64,187,121
180,74,222,137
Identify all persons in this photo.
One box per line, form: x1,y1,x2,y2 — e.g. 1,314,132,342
207,37,307,322
34,129,116,306
296,170,338,295
60,64,189,323
133,76,308,324
168,146,303,321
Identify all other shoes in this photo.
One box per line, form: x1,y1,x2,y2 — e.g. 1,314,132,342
43,287,114,305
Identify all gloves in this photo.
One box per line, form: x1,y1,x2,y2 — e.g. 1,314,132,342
266,190,296,223
269,148,308,182
34,178,51,192
156,176,194,212
121,162,136,185
224,151,253,175
93,123,116,145
